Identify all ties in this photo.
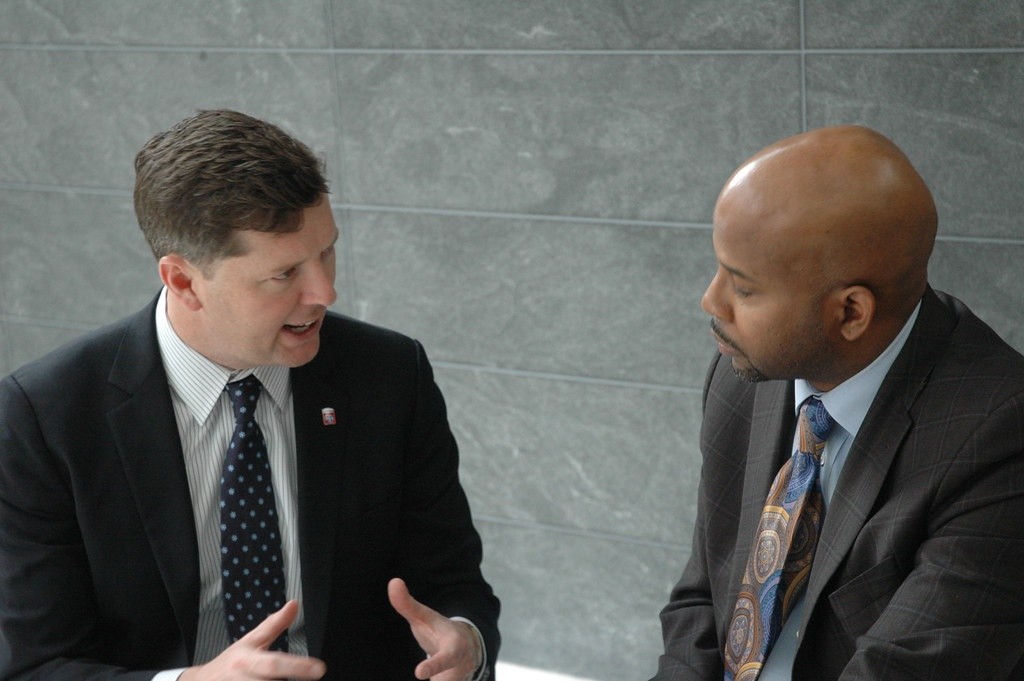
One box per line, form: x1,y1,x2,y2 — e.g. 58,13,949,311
220,375,292,656
724,397,835,681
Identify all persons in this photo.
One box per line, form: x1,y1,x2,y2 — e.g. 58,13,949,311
648,123,1023,681
0,109,501,681
